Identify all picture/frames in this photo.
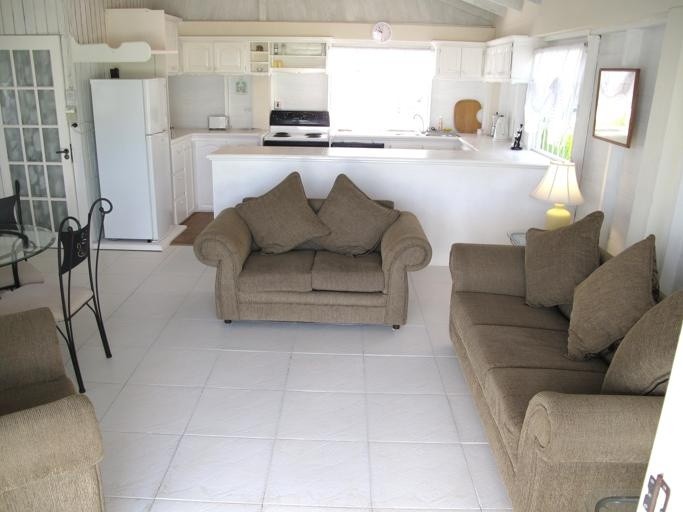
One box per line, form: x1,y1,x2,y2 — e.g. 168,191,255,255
592,67,641,150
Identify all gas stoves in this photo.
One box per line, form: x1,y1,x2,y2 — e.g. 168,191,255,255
262,131,329,146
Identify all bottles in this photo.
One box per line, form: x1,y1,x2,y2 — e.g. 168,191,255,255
439,115,443,130
511,124,523,151
490,112,500,136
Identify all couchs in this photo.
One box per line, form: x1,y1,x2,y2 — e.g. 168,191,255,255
447,241,668,511
192,197,433,330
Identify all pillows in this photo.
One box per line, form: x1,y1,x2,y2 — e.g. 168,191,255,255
599,286,682,396
523,209,605,310
233,171,331,258
565,233,661,363
292,173,402,259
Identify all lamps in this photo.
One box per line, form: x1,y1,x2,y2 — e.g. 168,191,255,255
527,160,587,232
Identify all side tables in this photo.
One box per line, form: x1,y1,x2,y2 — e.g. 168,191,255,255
505,229,527,247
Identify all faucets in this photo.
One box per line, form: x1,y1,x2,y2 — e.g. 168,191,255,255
413,114,426,135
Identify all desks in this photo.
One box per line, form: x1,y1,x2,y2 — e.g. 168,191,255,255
0,223,56,291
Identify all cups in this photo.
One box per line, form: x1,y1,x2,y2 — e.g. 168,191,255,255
274,43,278,54
280,43,287,55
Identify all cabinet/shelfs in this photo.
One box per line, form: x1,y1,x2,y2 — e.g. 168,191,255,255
170,135,195,225
269,36,333,73
484,34,532,85
195,136,261,203
384,140,455,150
249,36,270,76
429,41,484,80
181,36,249,76
104,7,184,79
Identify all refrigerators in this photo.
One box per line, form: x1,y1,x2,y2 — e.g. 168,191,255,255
90,77,175,244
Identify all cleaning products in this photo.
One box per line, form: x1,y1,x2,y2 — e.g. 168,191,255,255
488,112,509,140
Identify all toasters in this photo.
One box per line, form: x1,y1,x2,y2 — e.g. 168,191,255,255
208,115,229,130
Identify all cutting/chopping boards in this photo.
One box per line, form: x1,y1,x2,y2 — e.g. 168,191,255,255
453,98,482,135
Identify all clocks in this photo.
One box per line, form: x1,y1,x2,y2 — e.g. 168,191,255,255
370,21,394,45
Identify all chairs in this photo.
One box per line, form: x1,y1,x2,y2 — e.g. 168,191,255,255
0,306,107,512
0,179,44,290
0,197,113,394
